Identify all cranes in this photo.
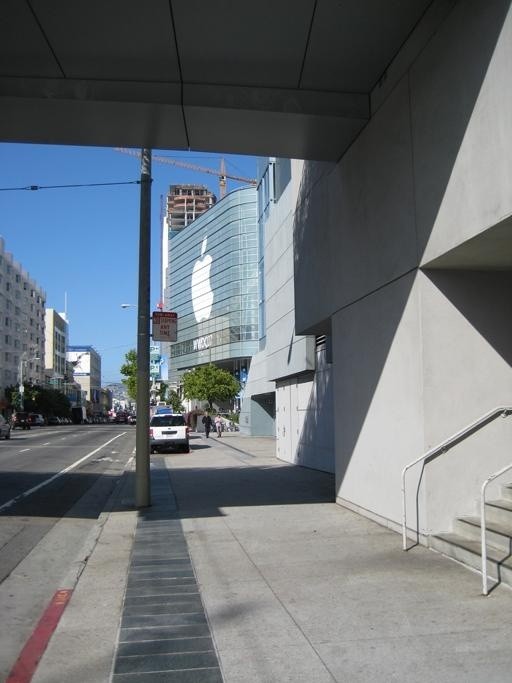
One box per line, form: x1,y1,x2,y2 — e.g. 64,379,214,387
113,147,256,199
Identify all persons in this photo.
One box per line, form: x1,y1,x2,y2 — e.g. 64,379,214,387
215,414,224,438
201,411,214,438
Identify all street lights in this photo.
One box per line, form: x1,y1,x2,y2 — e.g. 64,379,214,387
21,358,40,408
120,303,157,417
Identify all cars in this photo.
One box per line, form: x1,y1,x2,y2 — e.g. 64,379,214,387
0,411,73,439
149,401,189,453
129,416,136,425
